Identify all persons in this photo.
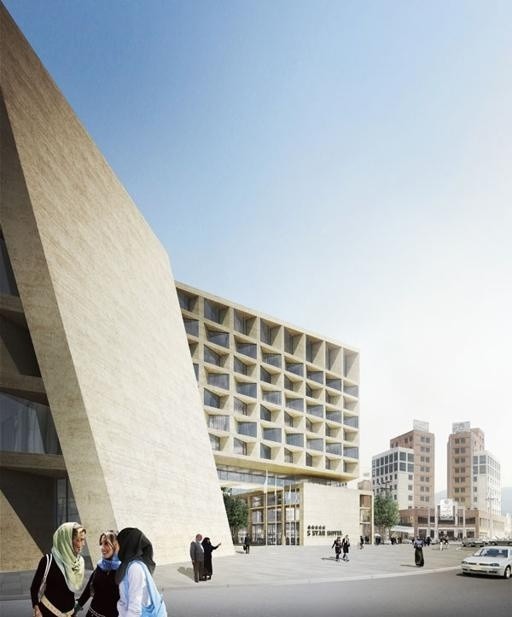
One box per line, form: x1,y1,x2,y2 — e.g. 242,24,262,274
30,522,86,617
201,537,221,580
332,537,342,561
245,533,251,554
342,535,350,561
115,528,167,617
190,534,206,582
76,530,123,617
360,536,448,567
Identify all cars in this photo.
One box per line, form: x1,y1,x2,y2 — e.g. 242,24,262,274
460,546,512,578
462,533,512,548
